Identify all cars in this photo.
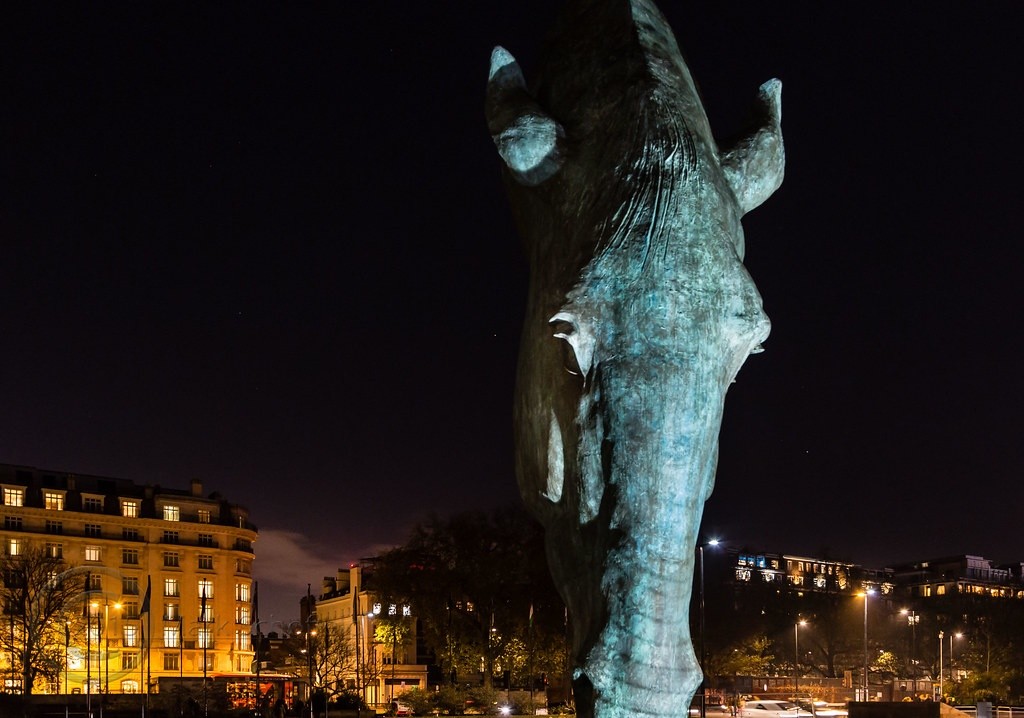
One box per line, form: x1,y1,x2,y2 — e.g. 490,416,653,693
738,699,815,718
391,700,416,717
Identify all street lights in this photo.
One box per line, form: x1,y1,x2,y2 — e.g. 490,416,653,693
367,612,396,702
864,588,876,701
794,621,807,718
950,632,963,681
699,539,720,718
908,610,919,694
491,627,497,687
307,631,317,718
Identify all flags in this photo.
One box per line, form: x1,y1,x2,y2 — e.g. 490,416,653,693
201,584,207,622
141,620,145,650
353,586,359,623
306,585,312,618
180,618,183,645
325,623,329,649
83,574,91,617
140,575,151,614
98,613,101,642
65,624,69,647
250,580,258,625
490,598,494,626
529,604,534,627
563,607,568,625
